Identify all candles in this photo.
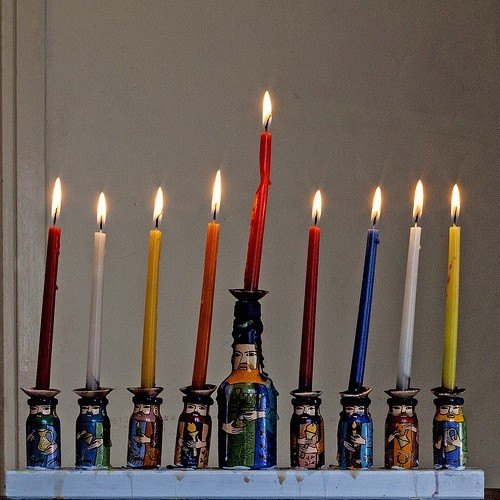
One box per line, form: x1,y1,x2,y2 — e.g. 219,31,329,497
395,181,423,390
35,176,62,388
348,184,381,392
140,184,164,387
192,169,222,390
439,183,462,389
244,89,274,290
85,191,108,391
299,190,322,391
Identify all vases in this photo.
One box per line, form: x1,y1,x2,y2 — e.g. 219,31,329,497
217,288,279,469
289,390,326,469
383,388,422,470
20,386,63,472
126,387,164,470
337,387,375,470
72,386,114,470
173,385,215,470
430,387,468,470
